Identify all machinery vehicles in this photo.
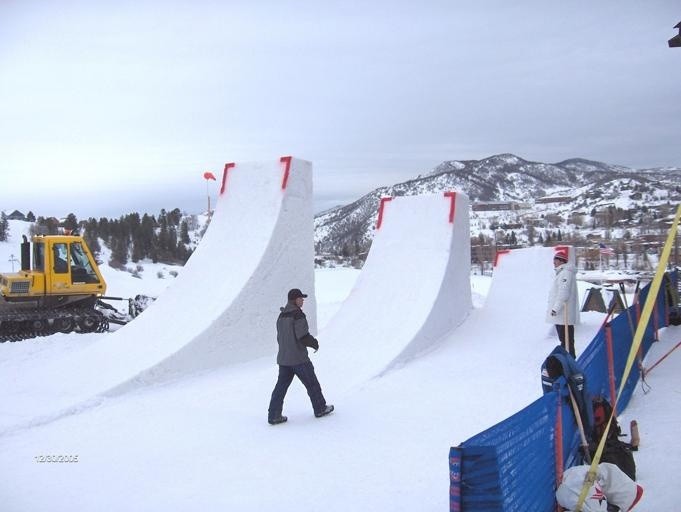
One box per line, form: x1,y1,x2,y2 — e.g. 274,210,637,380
0,235,157,344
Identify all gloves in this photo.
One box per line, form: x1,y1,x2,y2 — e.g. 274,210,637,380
311,339,319,349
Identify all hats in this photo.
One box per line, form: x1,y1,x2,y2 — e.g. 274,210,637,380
553,251,569,262
289,289,307,299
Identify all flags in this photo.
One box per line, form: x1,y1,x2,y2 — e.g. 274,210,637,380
600,242,616,256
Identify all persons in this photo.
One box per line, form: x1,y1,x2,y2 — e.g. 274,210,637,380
267,289,334,423
548,250,578,357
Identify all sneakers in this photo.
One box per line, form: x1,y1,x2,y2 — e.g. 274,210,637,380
269,415,288,424
315,405,334,417
